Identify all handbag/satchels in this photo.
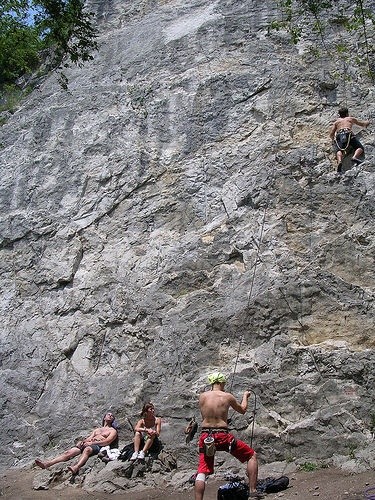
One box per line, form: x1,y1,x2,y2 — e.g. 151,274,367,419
218,481,248,500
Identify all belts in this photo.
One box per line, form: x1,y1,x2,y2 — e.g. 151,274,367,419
202,428,228,432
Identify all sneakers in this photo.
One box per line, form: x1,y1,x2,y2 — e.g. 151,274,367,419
138,450,145,459
248,491,267,500
130,452,138,460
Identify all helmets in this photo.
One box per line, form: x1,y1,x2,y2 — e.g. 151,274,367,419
208,372,226,384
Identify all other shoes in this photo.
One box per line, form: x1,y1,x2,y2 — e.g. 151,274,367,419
336,161,342,172
351,158,362,163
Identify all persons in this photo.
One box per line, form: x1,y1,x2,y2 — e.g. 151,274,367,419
35,412,118,474
129,402,161,461
329,107,370,172
194,372,264,500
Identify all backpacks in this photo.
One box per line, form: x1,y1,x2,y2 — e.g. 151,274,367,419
118,443,134,461
264,476,289,492
